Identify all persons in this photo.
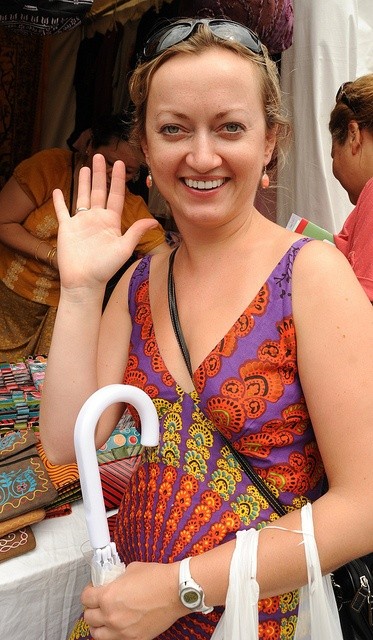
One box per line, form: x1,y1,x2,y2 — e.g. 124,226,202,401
325,74,373,302
1,111,172,365
38,14,373,640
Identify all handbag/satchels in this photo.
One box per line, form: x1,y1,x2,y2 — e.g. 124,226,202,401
332,557,373,640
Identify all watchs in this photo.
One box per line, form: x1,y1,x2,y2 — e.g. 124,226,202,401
177,557,213,614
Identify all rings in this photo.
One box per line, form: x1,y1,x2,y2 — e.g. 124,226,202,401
76,206,88,213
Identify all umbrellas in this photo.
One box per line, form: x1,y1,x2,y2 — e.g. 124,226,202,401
74,382,159,587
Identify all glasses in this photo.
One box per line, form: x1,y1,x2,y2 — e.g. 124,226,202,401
138,18,269,66
337,78,364,129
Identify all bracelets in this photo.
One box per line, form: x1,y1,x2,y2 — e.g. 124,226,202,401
45,247,55,267
33,240,52,262
49,249,56,267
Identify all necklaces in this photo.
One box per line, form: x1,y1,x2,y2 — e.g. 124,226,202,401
79,158,85,169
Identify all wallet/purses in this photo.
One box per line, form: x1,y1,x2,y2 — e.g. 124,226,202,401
0,432,81,565
